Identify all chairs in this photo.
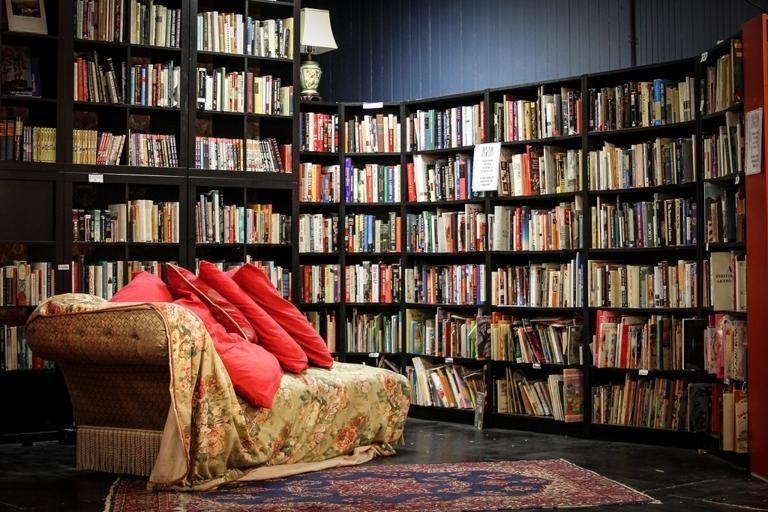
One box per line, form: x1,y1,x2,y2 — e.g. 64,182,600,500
24,293,411,481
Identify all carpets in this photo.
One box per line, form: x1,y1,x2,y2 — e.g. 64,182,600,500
103,455,665,512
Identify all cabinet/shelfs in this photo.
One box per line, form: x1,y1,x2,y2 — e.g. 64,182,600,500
1,0,300,384
299,26,750,466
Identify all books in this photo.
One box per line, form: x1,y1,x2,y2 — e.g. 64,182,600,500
298,37,749,453
1,0,293,370
